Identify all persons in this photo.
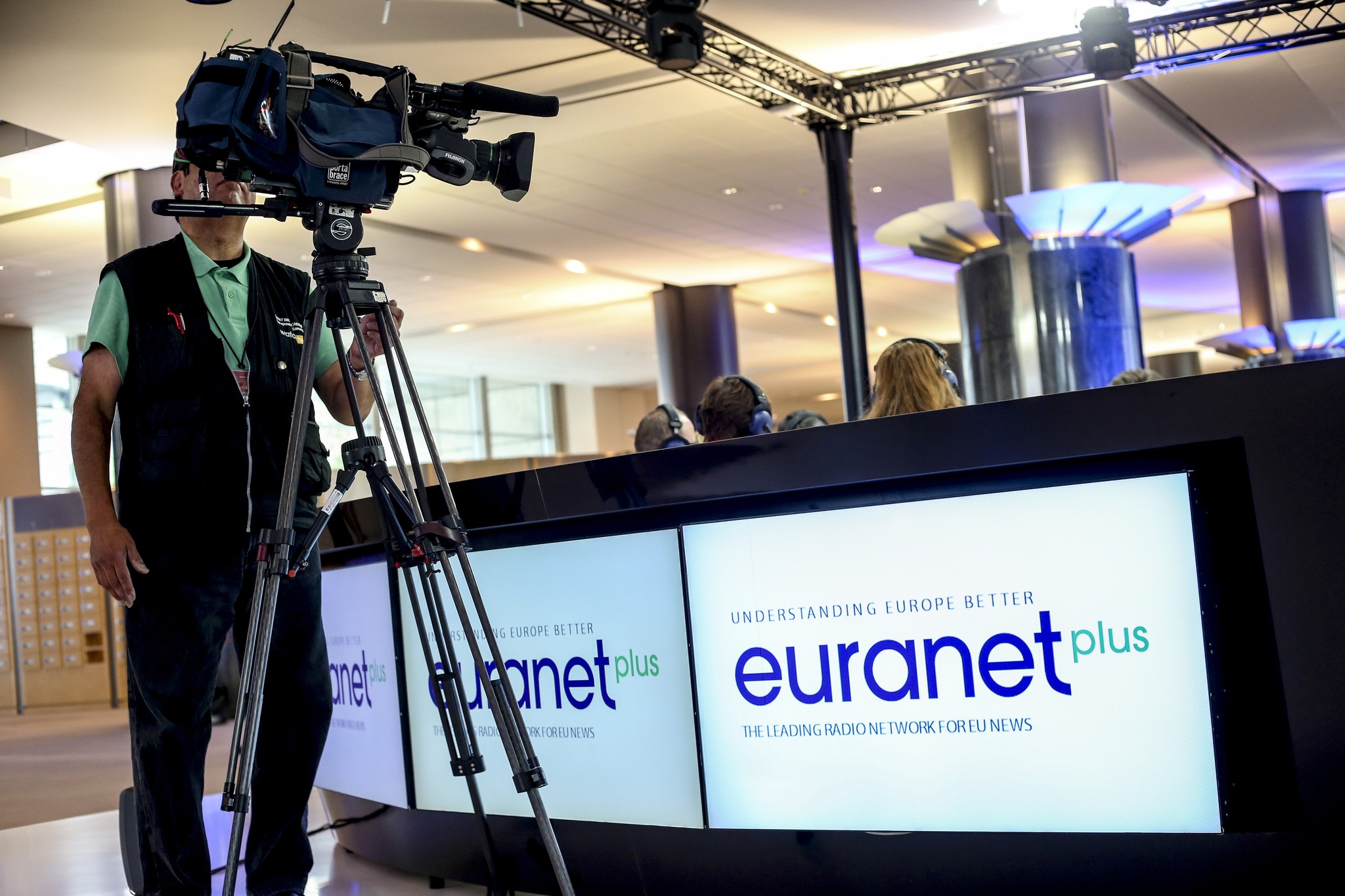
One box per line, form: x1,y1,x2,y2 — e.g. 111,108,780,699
776,410,830,434
634,403,695,454
70,148,403,896
694,375,772,443
860,338,967,419
1105,369,1167,387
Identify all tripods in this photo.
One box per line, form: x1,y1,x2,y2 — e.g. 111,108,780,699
151,197,574,896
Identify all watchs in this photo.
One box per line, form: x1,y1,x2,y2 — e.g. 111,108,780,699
347,349,375,381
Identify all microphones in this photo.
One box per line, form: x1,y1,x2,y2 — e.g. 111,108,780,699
463,81,559,117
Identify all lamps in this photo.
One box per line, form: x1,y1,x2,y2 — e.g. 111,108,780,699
645,0,706,70
1079,5,1137,79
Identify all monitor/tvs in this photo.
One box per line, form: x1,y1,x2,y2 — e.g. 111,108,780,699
311,466,1228,840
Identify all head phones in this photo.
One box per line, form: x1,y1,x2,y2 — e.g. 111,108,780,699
655,404,691,450
872,339,958,407
693,374,773,436
